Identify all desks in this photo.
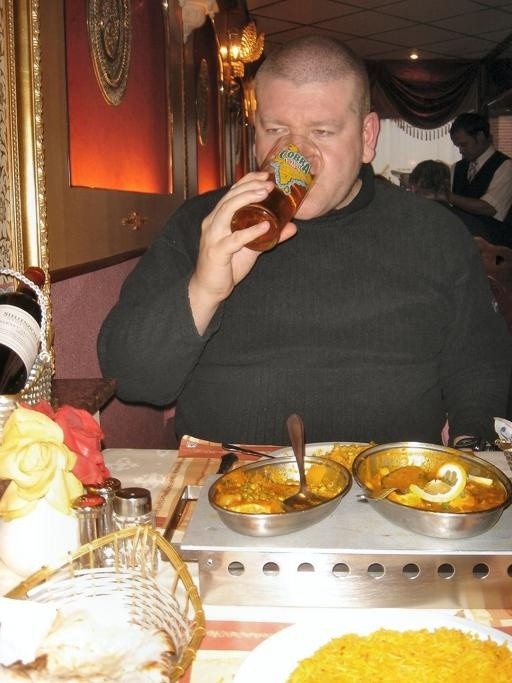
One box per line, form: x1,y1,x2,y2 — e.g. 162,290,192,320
0,377,122,507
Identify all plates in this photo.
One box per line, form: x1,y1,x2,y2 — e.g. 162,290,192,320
231,606,512,682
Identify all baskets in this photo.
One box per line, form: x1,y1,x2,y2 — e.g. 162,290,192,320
0,267,54,439
0,522,207,683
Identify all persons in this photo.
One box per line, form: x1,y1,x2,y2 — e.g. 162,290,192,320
407,111,512,250
406,159,490,242
95,34,512,452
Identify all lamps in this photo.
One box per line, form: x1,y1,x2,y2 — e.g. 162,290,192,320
228,19,267,79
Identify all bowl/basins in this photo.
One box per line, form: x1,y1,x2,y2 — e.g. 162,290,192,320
352,441,512,540
208,454,353,539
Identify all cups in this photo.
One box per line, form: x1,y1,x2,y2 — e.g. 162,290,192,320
229,133,325,252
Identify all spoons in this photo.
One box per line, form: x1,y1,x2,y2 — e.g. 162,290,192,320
280,412,323,514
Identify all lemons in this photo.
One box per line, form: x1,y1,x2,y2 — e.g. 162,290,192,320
407,462,468,503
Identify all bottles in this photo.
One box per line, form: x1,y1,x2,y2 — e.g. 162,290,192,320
70,476,159,578
0,265,46,395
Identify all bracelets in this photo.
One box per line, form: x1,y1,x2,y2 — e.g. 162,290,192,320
433,191,438,200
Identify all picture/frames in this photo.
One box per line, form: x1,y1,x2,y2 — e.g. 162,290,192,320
1,0,183,298
185,11,229,210
